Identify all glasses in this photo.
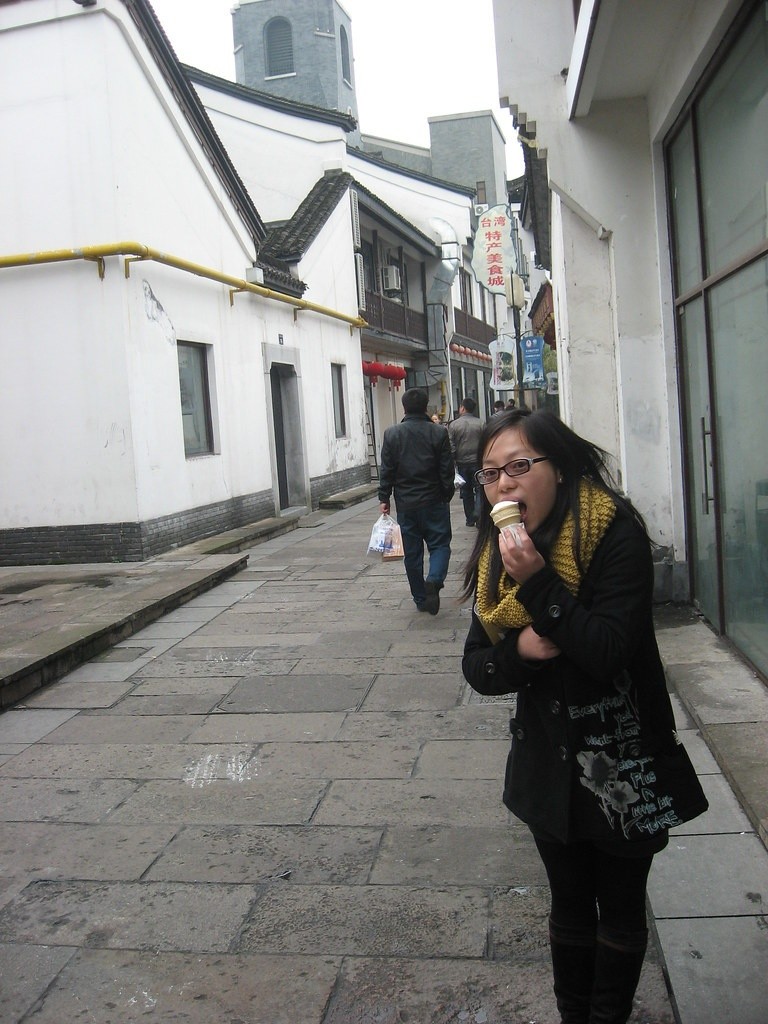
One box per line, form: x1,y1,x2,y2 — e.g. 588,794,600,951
474,456,549,485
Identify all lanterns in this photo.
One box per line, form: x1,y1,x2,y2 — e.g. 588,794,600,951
452,343,492,361
362,360,406,391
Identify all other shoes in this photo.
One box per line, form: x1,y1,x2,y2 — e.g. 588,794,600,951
466,520,475,526
418,605,425,612
424,581,444,615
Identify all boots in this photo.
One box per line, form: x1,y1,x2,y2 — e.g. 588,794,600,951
590,920,649,1024
548,913,599,1024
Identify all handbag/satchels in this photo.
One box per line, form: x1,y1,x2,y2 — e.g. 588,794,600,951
454,468,466,490
366,513,405,562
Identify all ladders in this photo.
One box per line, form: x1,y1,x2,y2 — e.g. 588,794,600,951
364,390,379,481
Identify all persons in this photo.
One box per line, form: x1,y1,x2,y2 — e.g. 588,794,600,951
431,413,440,423
492,401,505,416
462,409,710,1024
506,399,515,411
449,397,485,528
378,387,455,616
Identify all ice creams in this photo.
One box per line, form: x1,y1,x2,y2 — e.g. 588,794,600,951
490,501,522,549
